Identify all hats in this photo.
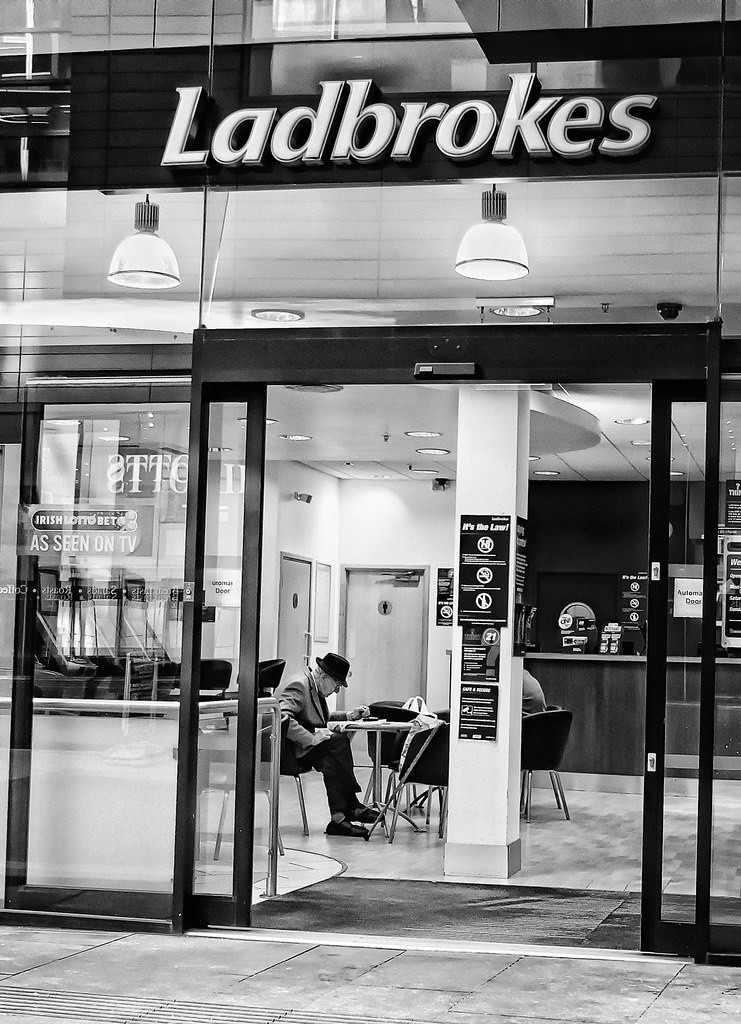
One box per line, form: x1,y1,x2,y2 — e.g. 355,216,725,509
316,653,350,687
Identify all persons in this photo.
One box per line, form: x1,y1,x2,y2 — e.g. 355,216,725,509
522,668,547,718
276,651,384,837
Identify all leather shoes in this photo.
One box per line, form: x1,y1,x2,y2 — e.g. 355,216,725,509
325,805,385,837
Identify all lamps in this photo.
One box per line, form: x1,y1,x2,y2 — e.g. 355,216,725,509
107,193,182,291
455,184,530,280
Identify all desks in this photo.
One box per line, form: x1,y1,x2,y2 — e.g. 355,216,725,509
324,720,428,838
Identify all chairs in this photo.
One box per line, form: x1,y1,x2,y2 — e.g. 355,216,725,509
522,710,574,823
174,658,232,699
174,700,464,861
521,702,563,814
237,658,286,697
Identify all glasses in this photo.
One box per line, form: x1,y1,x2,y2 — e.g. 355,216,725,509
327,678,341,687
